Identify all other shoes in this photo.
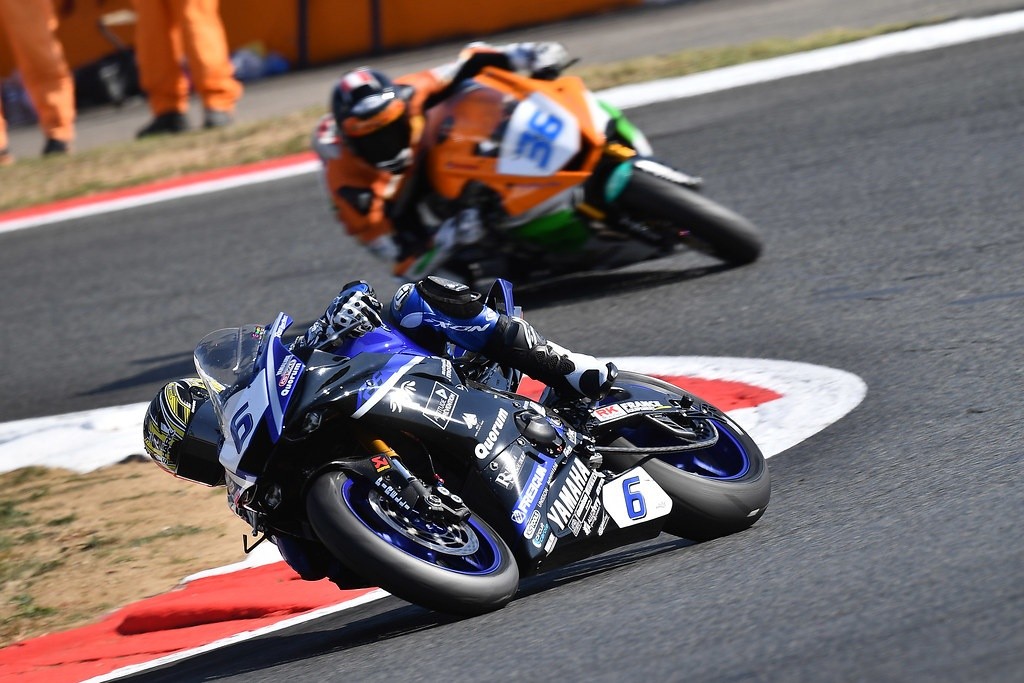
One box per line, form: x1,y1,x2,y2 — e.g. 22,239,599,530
43,139,66,154
136,111,188,138
0,149,14,167
204,111,230,129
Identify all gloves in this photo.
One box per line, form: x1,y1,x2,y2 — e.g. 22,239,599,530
324,284,391,340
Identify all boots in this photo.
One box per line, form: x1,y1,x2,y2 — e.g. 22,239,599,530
483,314,618,401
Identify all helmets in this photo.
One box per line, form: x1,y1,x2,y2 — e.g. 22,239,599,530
331,66,414,171
143,378,230,488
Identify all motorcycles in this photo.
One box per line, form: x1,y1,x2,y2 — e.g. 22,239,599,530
386,41,764,311
192,310,773,619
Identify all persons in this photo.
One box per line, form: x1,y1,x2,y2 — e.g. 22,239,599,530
130,0,244,138
0,0,76,159
325,35,648,283
143,278,620,577
